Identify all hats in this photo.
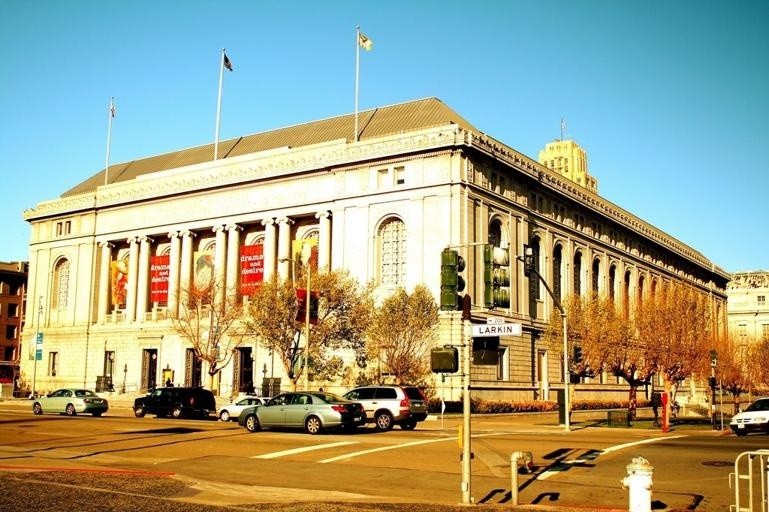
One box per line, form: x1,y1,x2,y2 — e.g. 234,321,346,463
217,395,274,422
238,391,367,434
32,388,110,417
729,397,769,436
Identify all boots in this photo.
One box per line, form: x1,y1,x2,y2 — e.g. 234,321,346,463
133,386,217,419
342,383,429,431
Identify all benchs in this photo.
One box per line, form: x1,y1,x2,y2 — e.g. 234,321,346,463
225,55,233,72
359,32,373,51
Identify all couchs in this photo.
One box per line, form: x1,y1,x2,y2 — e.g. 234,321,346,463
523,243,535,277
711,349,718,368
439,246,466,312
483,242,511,310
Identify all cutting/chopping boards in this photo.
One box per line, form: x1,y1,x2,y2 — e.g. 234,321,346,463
620,455,654,512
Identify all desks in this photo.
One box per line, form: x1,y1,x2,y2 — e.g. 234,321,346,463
278,257,315,393
